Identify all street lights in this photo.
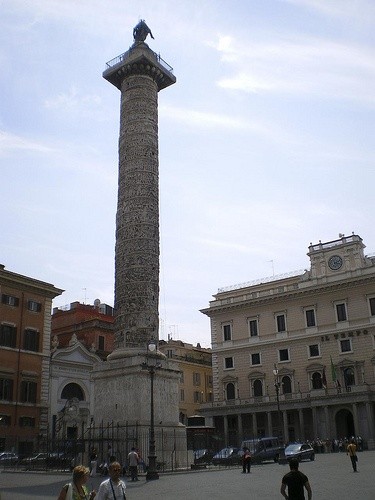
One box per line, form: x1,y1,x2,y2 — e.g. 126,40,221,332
140,335,162,481
272,363,288,465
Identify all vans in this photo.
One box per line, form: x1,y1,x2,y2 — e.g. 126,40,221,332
238,436,279,466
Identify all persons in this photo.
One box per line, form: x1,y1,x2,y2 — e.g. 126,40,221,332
127,447,142,481
241,445,252,473
346,440,358,472
107,445,116,462
89,448,98,478
293,436,363,453
280,458,312,500
57,465,96,500
94,462,129,500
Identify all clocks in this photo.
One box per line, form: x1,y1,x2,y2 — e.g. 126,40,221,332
328,255,342,270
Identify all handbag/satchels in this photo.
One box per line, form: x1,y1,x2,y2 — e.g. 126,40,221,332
354,455,358,461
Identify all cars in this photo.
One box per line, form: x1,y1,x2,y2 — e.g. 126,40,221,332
0,452,18,464
277,442,315,464
212,447,241,466
23,452,64,465
